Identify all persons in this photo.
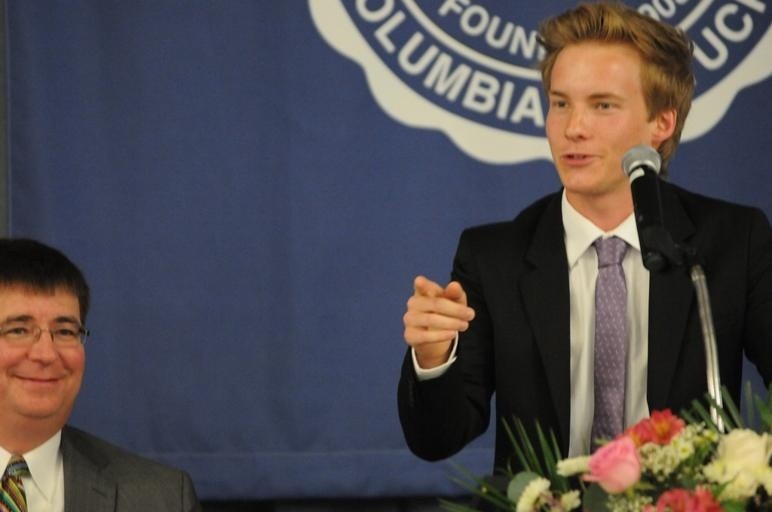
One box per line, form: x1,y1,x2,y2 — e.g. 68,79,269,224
0,235,198,511
398,4,772,511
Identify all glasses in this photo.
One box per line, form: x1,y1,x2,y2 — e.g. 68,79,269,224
1,316,91,347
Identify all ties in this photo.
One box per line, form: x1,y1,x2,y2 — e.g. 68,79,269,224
0,454,29,512
590,237,627,455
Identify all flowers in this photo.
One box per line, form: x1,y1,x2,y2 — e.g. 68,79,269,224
437,379,772,512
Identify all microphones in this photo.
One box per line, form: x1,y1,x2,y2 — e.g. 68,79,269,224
621,143,668,273
